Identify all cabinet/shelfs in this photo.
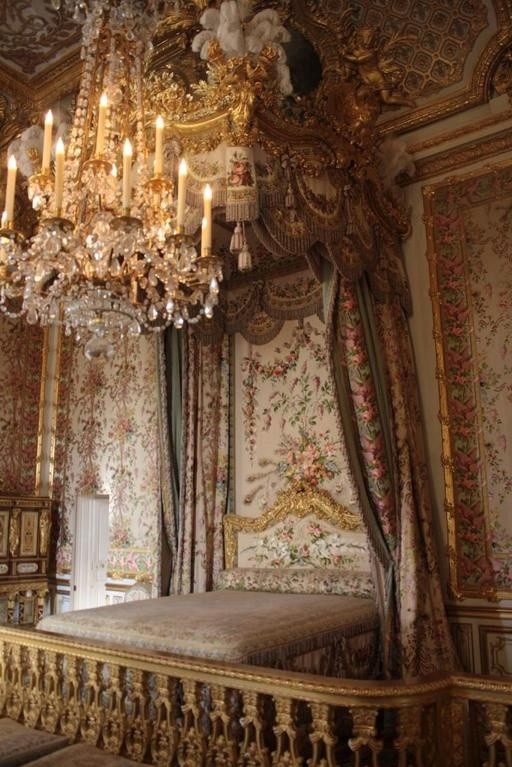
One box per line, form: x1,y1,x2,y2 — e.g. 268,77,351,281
0,492,57,629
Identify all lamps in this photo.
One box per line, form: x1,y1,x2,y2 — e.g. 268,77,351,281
0,2,224,363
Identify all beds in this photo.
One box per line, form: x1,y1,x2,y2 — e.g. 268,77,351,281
36,488,391,722
